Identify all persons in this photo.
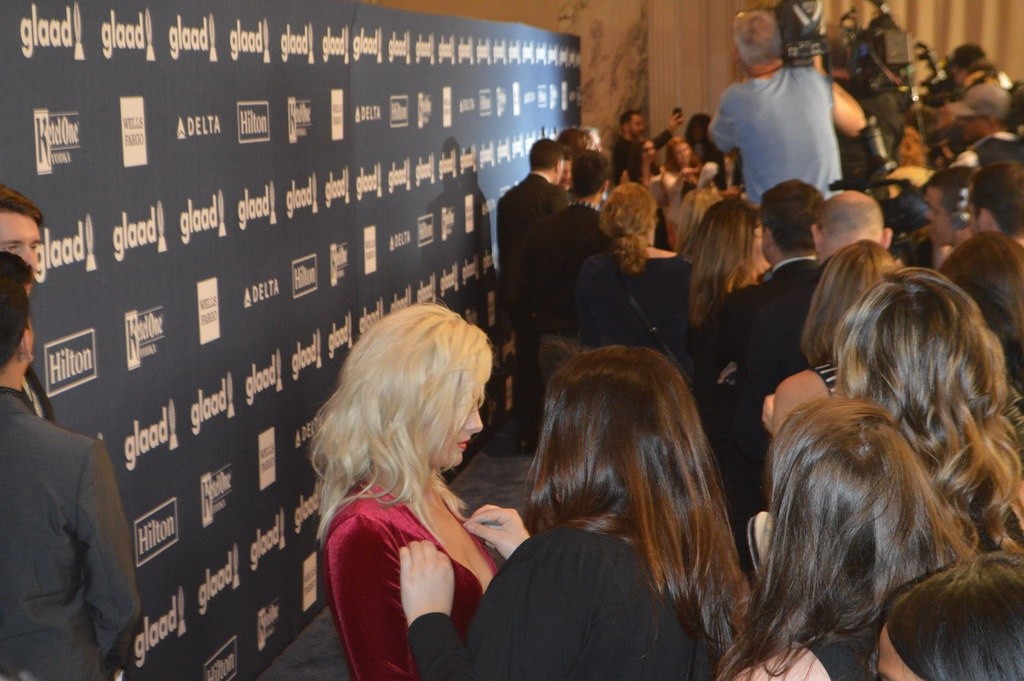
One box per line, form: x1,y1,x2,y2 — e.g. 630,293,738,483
496,140,574,456
876,556,1024,680
556,44,1024,581
0,250,32,301
0,277,139,681
0,185,57,428
715,395,977,681
399,345,745,681
707,9,868,209
309,298,498,681
518,149,615,391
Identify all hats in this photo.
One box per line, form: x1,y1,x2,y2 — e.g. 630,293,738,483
945,83,1013,118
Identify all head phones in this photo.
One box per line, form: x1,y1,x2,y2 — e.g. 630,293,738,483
948,183,971,229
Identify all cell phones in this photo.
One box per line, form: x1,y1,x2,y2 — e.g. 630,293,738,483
673,108,681,124
